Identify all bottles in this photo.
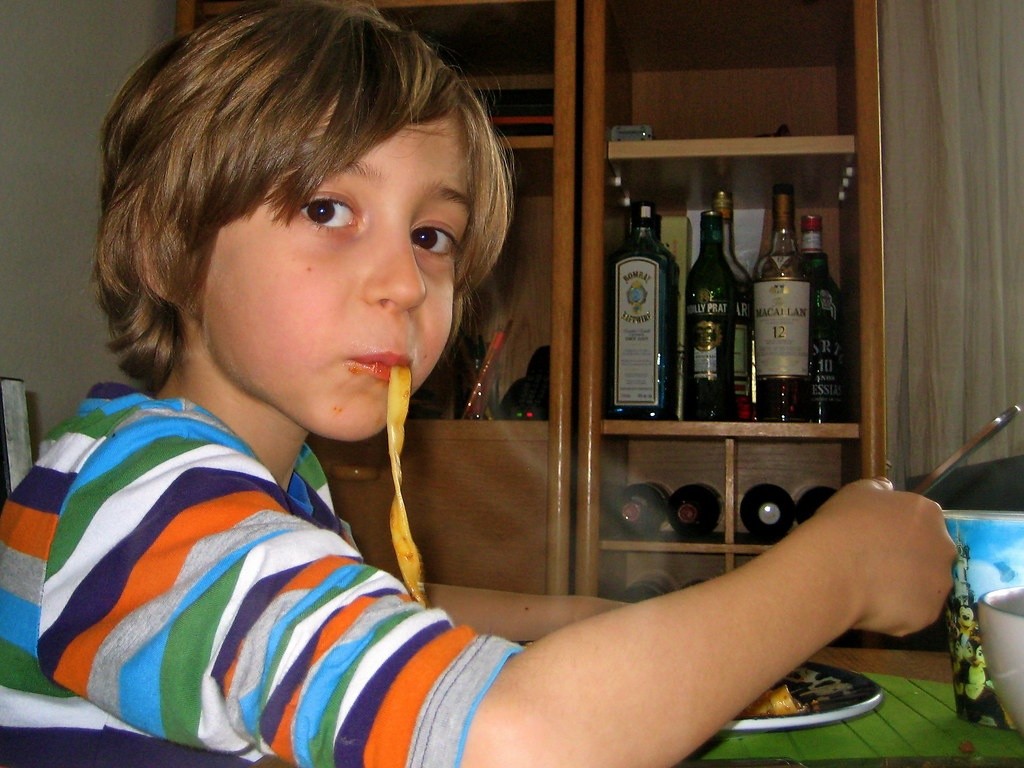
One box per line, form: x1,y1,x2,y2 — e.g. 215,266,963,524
739,483,795,540
666,484,725,535
611,185,847,422
793,484,837,525
615,483,667,534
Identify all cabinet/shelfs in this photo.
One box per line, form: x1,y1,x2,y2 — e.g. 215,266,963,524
574,0,885,606
175,0,576,595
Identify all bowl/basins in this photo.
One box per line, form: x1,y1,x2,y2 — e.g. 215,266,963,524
979,586,1024,738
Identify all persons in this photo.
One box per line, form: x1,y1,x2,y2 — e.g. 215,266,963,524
0,0,958,768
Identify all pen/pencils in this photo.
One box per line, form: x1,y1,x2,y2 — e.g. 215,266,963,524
449,316,515,420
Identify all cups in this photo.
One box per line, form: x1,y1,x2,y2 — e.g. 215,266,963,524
453,362,500,420
937,510,1024,732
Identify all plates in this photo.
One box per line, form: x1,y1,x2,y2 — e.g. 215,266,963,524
724,660,884,730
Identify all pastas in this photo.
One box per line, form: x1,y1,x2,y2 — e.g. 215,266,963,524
740,683,806,717
386,366,422,619
